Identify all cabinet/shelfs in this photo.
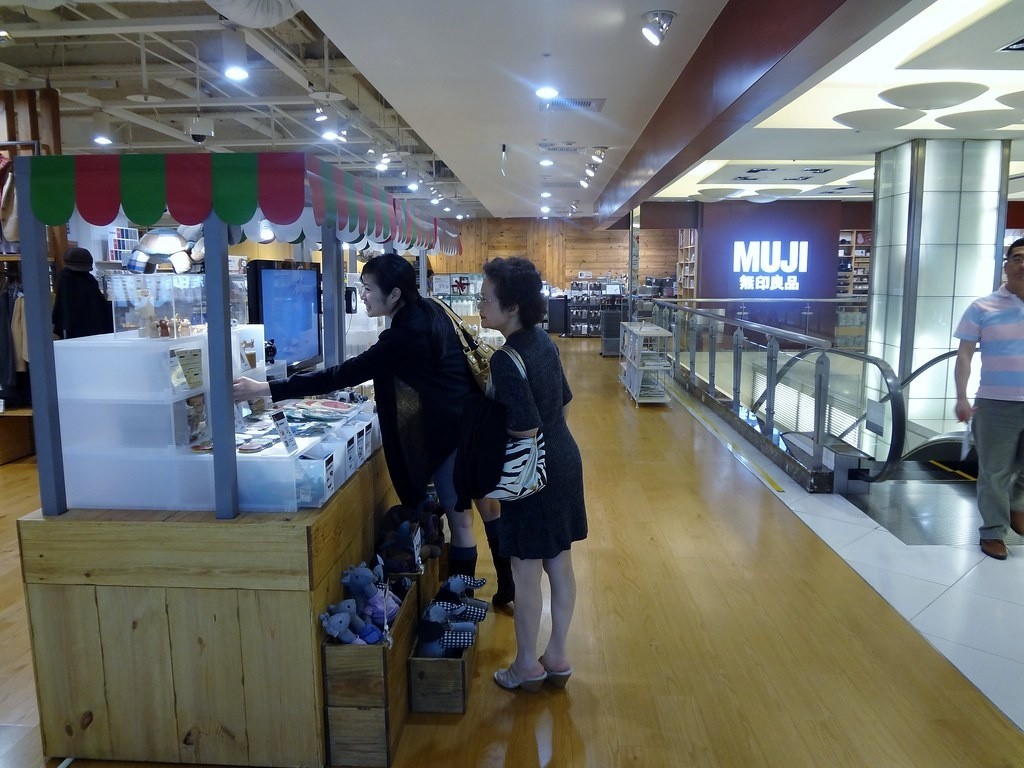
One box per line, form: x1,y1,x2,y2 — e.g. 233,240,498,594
619,321,674,409
677,229,698,320
431,271,505,346
838,229,873,293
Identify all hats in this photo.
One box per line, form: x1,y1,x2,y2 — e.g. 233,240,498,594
64,247,93,271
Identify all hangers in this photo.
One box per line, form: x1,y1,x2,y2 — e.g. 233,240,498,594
0,270,25,298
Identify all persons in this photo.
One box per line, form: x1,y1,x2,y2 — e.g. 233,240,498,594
233,255,517,608
478,256,587,695
952,238,1024,560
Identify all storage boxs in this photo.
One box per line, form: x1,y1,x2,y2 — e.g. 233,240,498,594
322,551,479,768
53,273,383,512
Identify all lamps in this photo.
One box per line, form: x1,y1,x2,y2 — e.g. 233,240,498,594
585,163,601,177
336,122,352,142
312,97,327,123
220,29,248,82
639,10,677,46
580,176,591,188
92,112,112,145
366,144,445,205
591,147,606,163
568,201,582,216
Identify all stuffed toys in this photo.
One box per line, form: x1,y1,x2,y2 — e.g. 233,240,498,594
318,503,490,659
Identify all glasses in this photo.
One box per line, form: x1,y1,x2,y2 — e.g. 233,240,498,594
480,296,497,303
1009,258,1024,264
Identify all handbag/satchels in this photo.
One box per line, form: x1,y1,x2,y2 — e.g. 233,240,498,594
485,428,547,501
466,343,498,393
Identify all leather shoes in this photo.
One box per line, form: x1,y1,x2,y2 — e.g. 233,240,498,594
1010,509,1024,534
980,538,1007,560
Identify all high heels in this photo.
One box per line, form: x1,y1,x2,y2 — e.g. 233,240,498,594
494,661,547,693
538,655,573,688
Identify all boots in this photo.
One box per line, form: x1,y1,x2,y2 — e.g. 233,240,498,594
483,517,515,606
448,545,477,599
